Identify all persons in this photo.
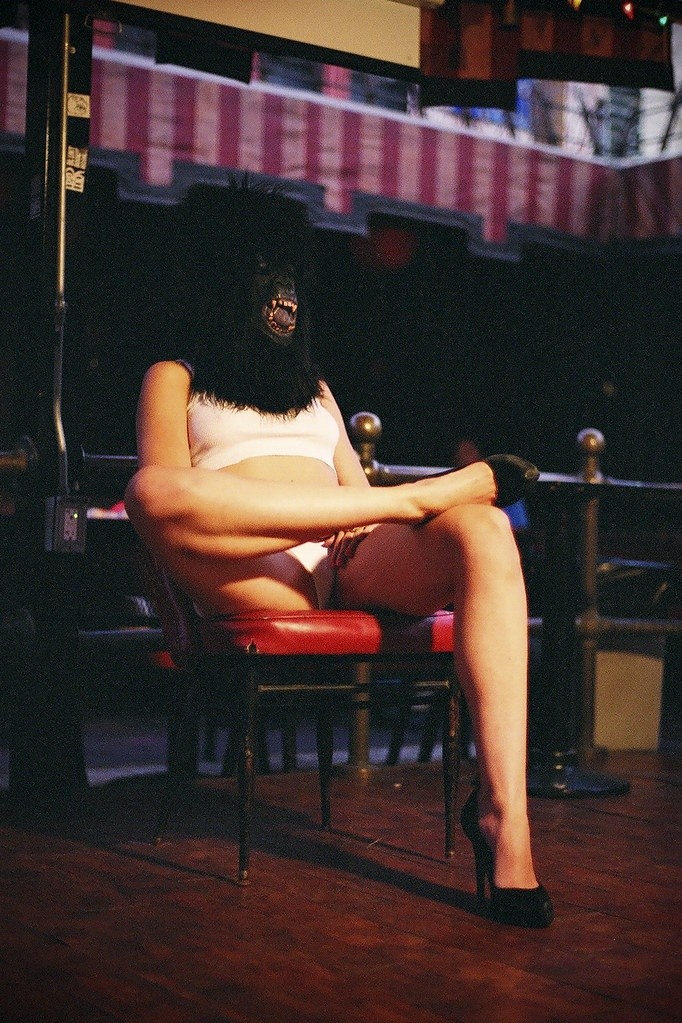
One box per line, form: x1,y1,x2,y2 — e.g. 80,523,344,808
123,182,554,929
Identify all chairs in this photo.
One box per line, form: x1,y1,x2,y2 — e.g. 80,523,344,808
125,518,466,884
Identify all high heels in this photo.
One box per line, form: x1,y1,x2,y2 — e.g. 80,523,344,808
406,454,539,511
459,786,553,929
